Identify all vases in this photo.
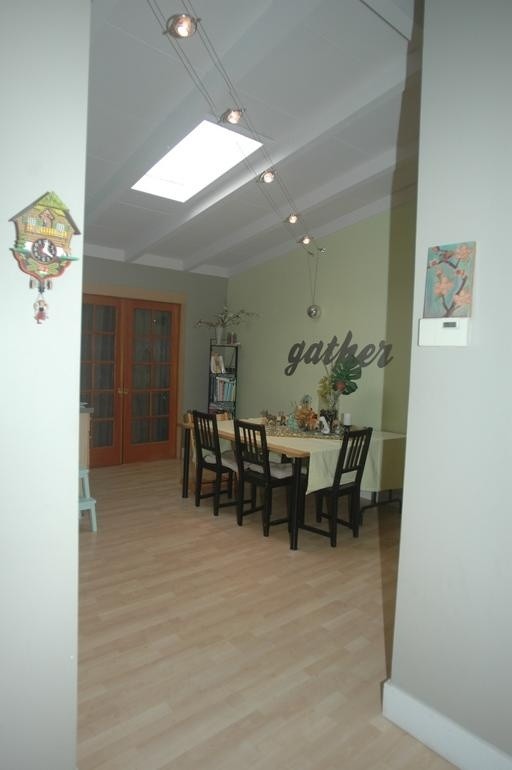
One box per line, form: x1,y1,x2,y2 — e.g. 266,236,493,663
216,326,222,344
320,409,337,433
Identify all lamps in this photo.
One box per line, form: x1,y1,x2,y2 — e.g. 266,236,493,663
147,0,314,248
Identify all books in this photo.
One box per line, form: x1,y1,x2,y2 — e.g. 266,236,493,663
209,352,236,420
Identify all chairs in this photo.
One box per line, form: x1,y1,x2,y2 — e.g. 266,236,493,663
78,469,97,531
299,426,373,547
234,420,295,537
192,410,257,516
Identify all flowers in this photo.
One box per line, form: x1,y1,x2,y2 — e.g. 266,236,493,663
191,302,260,329
317,355,362,411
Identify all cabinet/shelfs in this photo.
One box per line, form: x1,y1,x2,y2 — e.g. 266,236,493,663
208,344,240,419
